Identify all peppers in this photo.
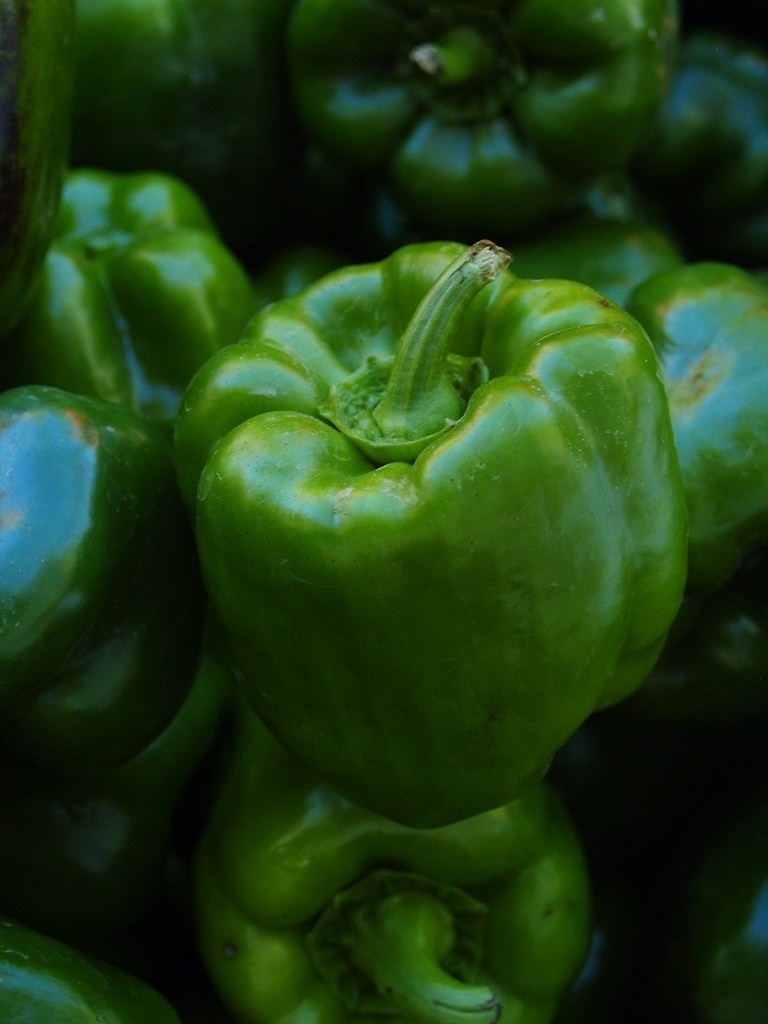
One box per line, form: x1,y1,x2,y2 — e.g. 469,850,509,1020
1,2,768,1024
